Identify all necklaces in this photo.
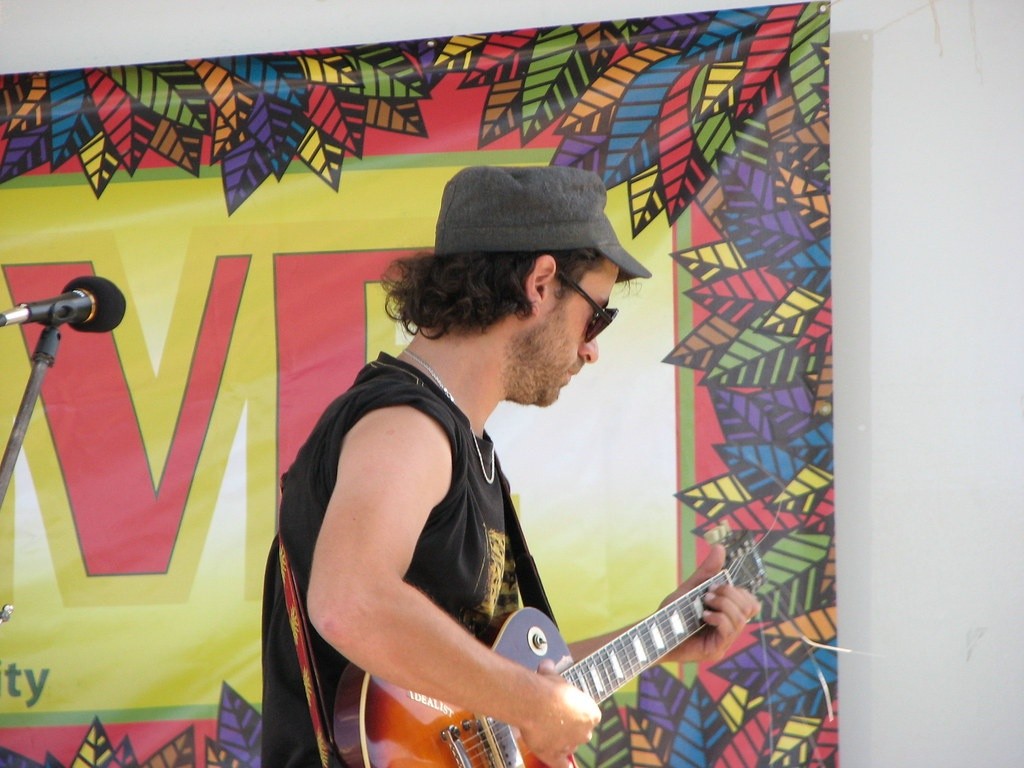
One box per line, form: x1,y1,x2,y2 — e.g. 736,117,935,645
403,350,494,484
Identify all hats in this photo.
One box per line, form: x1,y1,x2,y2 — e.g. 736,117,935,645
436,165,654,282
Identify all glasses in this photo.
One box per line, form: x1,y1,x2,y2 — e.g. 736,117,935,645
556,268,616,342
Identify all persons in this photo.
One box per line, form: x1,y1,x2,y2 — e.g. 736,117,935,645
263,164,765,768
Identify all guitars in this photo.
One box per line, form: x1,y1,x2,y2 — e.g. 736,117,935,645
333,520,765,768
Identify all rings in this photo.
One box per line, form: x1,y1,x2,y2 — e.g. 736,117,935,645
746,618,752,625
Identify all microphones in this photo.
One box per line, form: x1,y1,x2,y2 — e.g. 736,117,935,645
0,276,126,333
